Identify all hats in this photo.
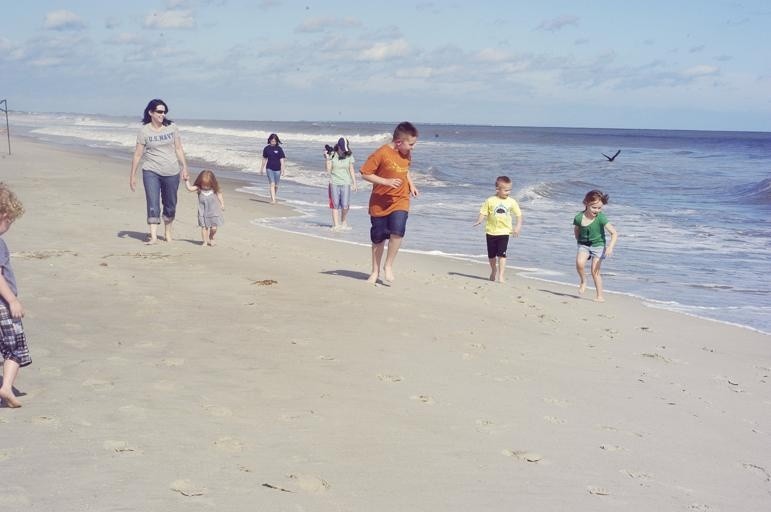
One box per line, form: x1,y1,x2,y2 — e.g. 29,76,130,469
338,137,350,152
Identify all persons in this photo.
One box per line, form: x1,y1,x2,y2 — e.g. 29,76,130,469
571,189,621,301
472,176,523,285
129,99,191,245
181,168,226,248
1,180,34,411
357,121,421,286
260,133,288,205
323,137,359,229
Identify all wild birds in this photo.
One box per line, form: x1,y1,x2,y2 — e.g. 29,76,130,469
601,150,621,161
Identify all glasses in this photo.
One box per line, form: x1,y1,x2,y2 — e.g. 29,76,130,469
155,110,167,114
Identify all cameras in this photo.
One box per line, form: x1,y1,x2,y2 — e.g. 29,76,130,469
325,145,333,154
